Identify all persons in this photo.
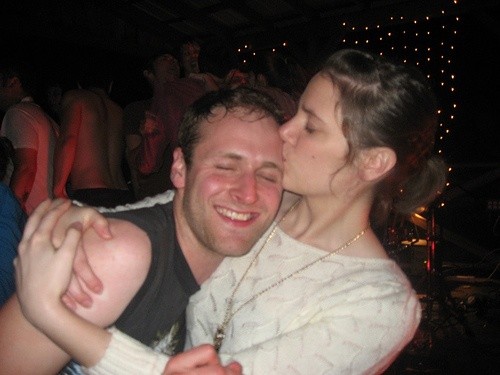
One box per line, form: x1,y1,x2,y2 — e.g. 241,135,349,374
0,38,302,305
0,89,282,374
11,47,448,373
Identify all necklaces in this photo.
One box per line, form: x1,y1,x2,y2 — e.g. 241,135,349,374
214,196,372,353
